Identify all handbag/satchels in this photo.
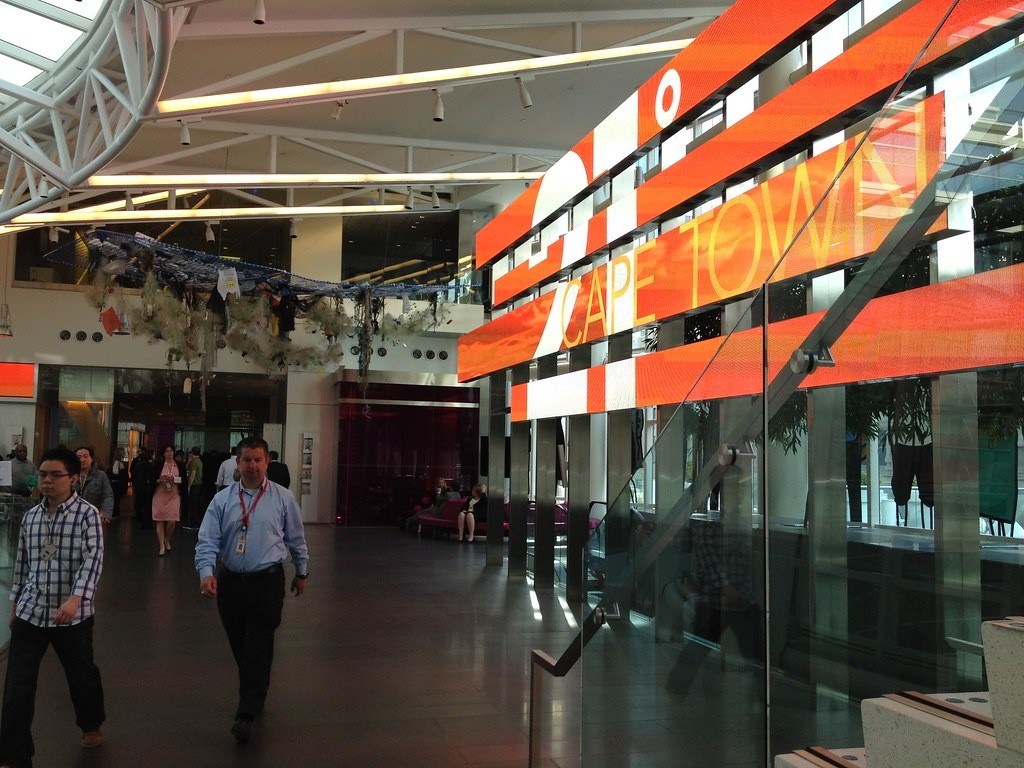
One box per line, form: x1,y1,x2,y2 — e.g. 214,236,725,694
105,460,121,480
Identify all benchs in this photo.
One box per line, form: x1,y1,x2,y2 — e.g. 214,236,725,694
417,499,600,538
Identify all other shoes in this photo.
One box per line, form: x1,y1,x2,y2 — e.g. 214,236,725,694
165,539,172,550
187,525,200,530
159,546,165,554
467,537,475,543
0,765,12,768
457,537,464,543
665,664,685,694
231,719,256,743
183,525,191,528
81,726,104,747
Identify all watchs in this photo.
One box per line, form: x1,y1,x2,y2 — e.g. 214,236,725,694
295,571,309,580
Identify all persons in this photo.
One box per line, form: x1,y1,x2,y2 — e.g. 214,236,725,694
193,436,313,738
0,448,108,768
404,476,462,530
1,442,291,557
661,462,783,708
457,483,489,544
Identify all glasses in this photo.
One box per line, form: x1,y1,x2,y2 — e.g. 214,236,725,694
36,473,72,480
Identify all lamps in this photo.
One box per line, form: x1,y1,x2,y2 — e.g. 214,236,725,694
432,189,440,209
328,103,343,121
38,174,48,198
519,82,532,109
405,190,414,209
51,231,58,243
433,94,444,122
206,225,215,243
180,126,190,145
290,223,297,238
253,0,265,25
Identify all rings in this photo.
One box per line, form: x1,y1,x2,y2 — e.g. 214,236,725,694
200,591,205,594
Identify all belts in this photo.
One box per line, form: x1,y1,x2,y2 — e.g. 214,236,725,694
219,564,283,583
112,480,120,482
